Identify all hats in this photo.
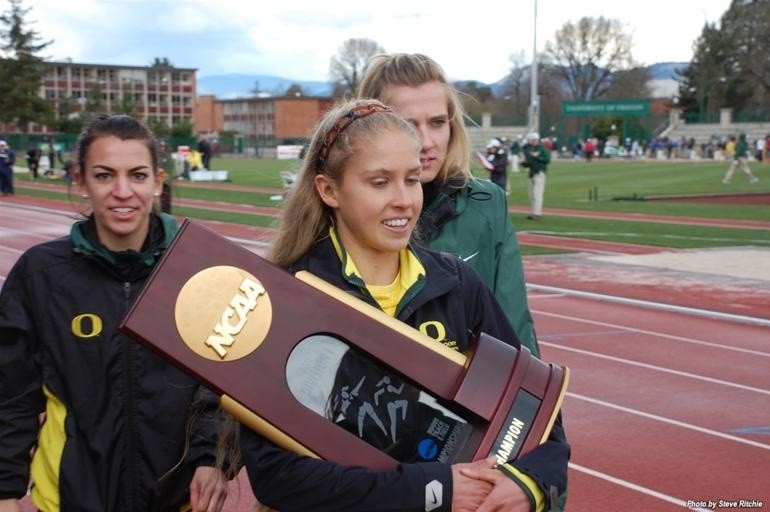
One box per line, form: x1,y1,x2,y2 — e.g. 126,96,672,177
486,139,500,149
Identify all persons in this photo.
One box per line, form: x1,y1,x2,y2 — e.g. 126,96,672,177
473,124,770,221
0,115,245,512
242,99,570,512
356,53,538,362
0,135,213,198
